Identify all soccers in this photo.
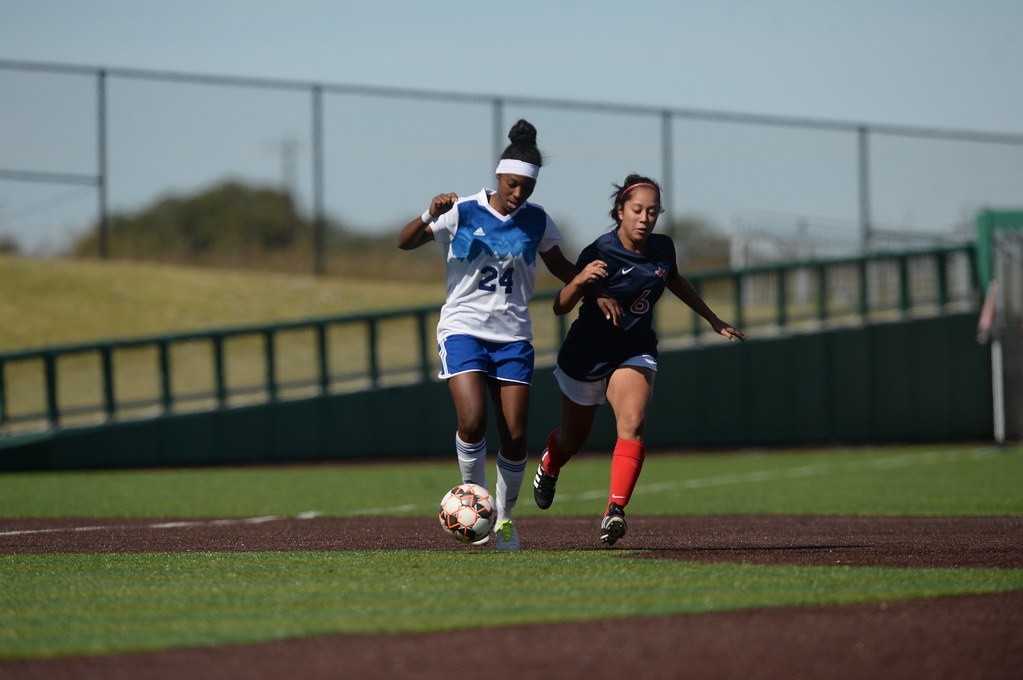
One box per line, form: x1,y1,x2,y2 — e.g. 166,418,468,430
439,482,495,540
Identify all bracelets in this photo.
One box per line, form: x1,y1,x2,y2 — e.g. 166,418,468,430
421,210,438,225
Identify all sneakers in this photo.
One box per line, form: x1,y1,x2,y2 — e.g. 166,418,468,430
600,501,627,546
471,534,490,546
533,448,560,511
493,517,520,552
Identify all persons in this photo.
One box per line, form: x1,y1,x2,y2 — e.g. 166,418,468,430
397,119,627,553
533,174,747,547
